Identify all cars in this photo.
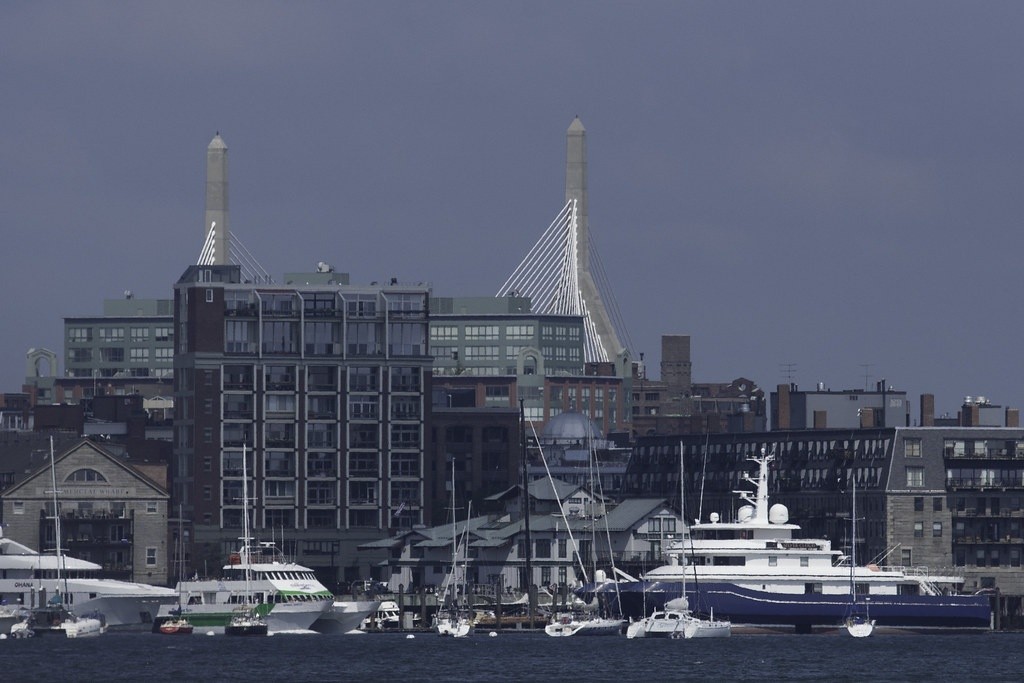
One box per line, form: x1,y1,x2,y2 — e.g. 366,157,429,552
348,579,372,594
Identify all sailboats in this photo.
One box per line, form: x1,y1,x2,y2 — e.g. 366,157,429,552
626,440,731,641
160,505,193,635
844,473,876,637
19,435,76,633
225,442,269,636
474,398,553,627
435,457,475,638
545,416,628,636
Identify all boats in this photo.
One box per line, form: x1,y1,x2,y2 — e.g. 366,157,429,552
573,449,990,632
360,601,421,631
308,600,382,636
65,614,108,639
152,537,334,634
0,525,180,631
